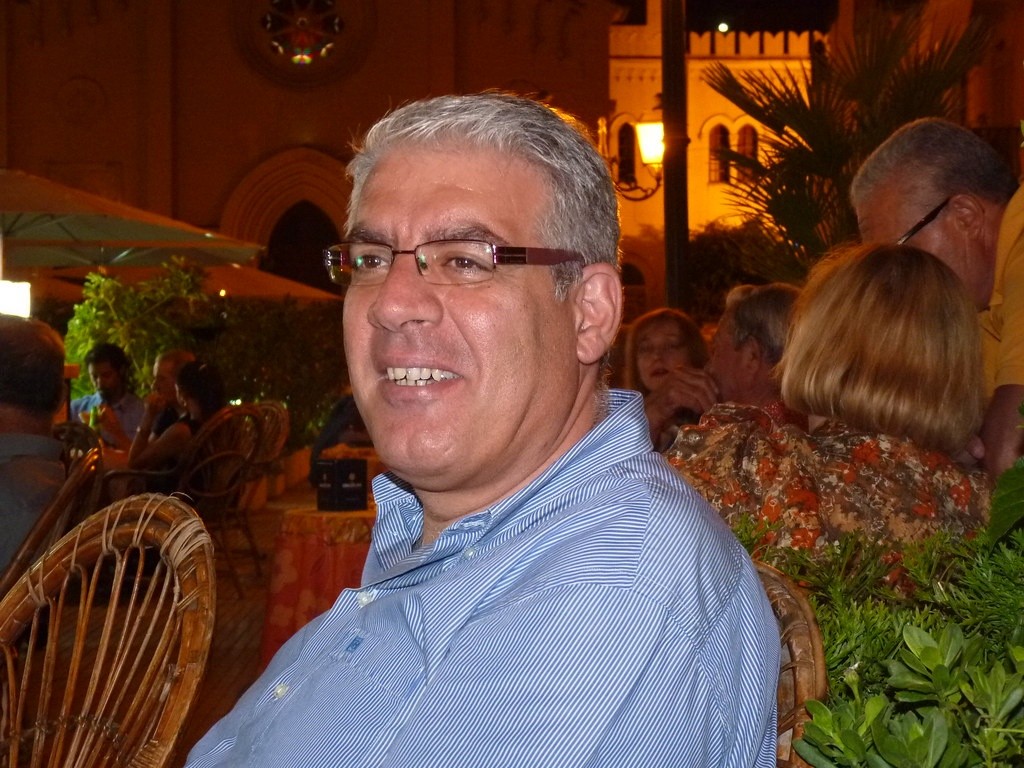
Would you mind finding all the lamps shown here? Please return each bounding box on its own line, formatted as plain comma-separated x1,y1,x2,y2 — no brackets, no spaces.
597,117,665,203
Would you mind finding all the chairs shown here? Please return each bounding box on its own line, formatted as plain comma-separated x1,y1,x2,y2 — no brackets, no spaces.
200,402,290,576
101,405,265,599
0,493,216,768
0,446,104,602
748,557,830,768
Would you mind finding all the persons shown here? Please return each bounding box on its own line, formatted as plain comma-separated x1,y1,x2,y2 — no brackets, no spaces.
175,85,783,768
0,313,230,584
622,108,1023,597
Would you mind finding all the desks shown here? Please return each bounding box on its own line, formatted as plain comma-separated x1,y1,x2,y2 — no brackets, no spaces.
255,502,377,675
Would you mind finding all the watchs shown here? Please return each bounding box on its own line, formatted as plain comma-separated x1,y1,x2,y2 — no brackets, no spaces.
136,425,150,437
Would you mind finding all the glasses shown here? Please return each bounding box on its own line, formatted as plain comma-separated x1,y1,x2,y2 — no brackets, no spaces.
322,239,582,288
